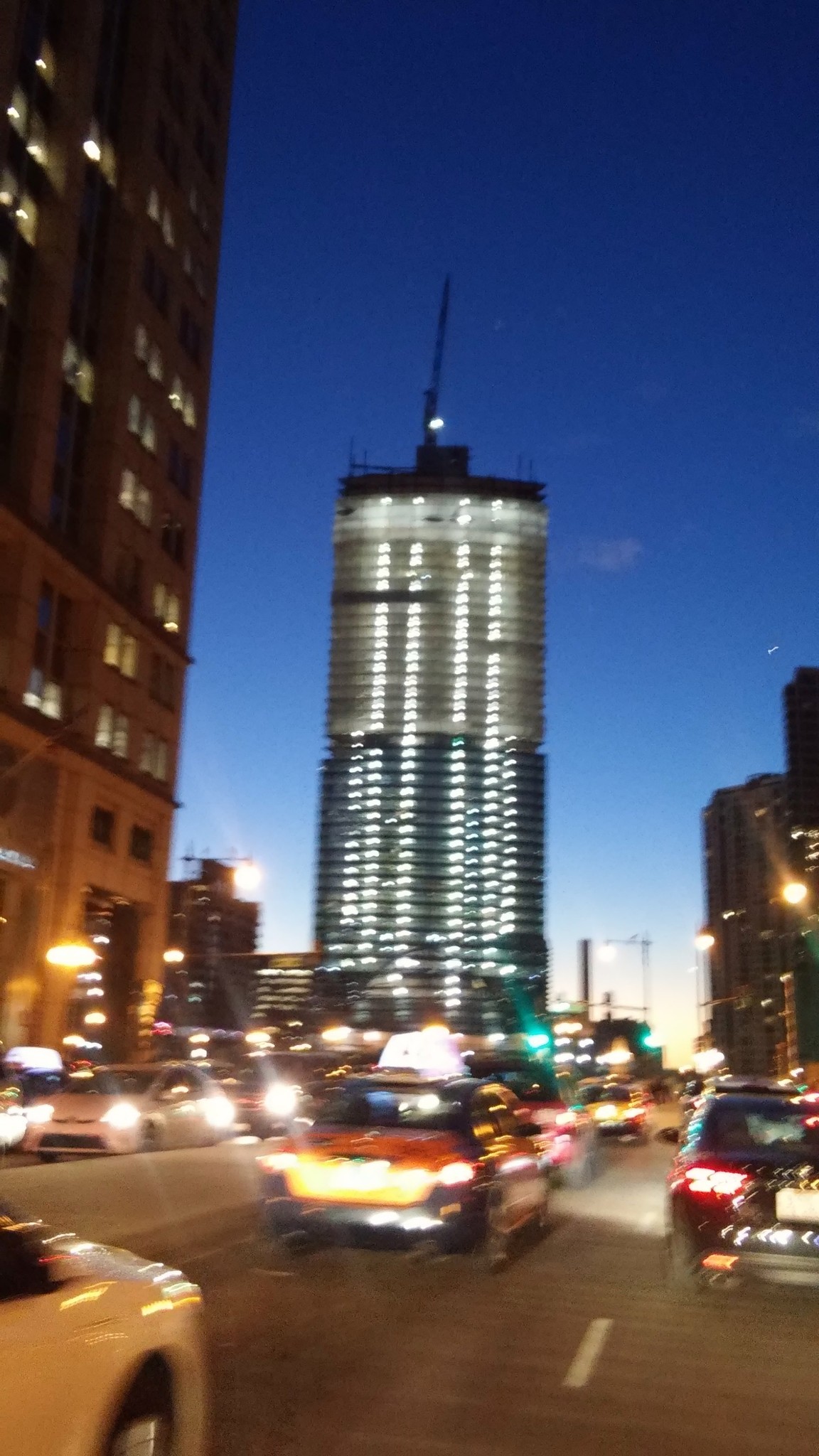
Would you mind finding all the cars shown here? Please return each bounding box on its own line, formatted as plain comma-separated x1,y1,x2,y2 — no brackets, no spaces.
21,1063,235,1161
2,1045,64,1088
150,1010,818,1167
457,1049,600,1189
258,1064,552,1260
0,1195,210,1456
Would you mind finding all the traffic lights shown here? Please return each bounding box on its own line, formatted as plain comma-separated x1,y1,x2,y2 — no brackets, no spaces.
523,1029,551,1054
642,1034,663,1051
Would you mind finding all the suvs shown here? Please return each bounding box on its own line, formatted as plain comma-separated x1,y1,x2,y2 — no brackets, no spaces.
652,1085,819,1294
